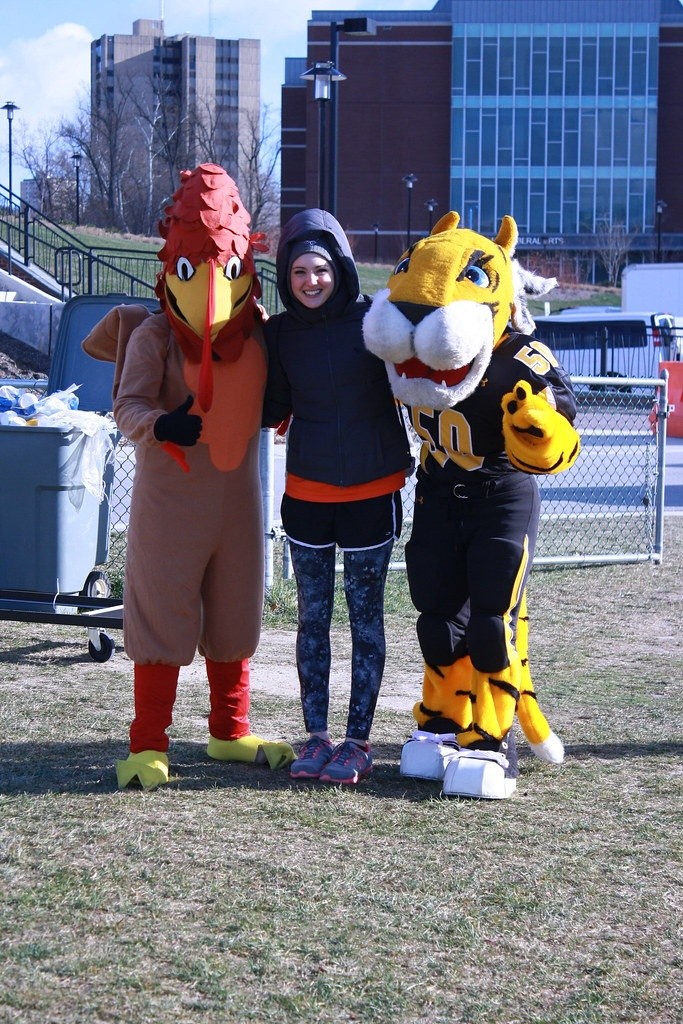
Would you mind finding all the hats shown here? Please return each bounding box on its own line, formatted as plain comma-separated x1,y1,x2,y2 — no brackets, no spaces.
287,238,339,310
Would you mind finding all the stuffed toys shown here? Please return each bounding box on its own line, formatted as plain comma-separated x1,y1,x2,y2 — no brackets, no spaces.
81,162,296,792
362,211,580,799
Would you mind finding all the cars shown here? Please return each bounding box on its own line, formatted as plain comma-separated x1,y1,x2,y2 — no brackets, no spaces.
550,305,622,314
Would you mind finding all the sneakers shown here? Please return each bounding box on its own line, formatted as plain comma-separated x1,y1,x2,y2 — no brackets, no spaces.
290,736,336,778
320,742,374,784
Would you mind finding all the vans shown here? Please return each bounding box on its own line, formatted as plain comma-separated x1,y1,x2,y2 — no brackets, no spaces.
510,312,677,394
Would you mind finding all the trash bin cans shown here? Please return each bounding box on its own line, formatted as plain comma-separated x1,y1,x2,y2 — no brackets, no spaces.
0,293,159,614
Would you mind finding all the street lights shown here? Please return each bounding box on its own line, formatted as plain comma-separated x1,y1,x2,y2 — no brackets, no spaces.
401,172,419,249
424,199,439,235
654,200,668,263
0,101,20,215
70,154,83,225
371,224,382,262
299,60,347,212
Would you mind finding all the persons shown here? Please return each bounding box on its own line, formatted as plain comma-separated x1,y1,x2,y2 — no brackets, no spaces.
261,210,418,784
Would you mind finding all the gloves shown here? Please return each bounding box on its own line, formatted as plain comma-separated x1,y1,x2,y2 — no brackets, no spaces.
154,395,203,446
